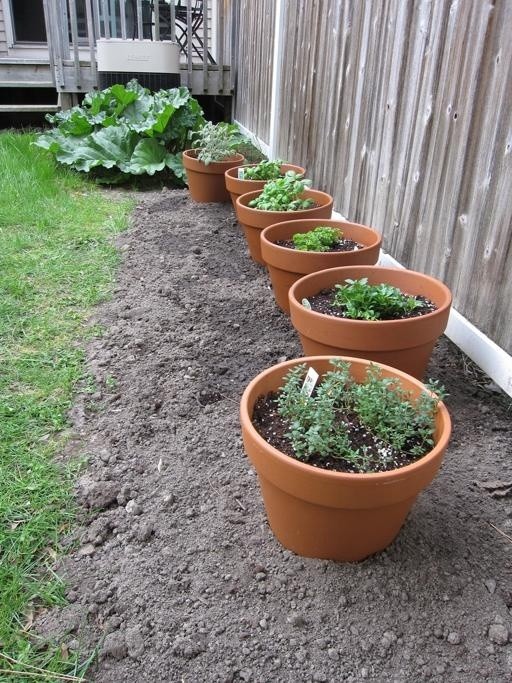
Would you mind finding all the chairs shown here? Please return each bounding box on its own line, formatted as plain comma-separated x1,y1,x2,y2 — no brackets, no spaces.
130,0,216,64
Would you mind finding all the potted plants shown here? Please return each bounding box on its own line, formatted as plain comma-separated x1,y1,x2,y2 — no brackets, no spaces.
260,218,381,316
288,265,453,382
183,122,252,204
225,161,304,217
236,171,333,264
239,355,452,563
